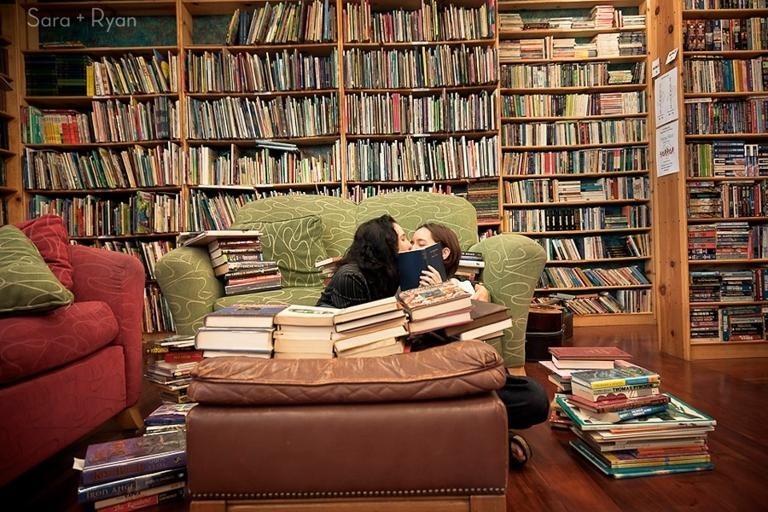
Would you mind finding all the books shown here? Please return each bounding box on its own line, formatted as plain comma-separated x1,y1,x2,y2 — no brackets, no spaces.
535,345,720,479
14,0,766,363
69,333,206,512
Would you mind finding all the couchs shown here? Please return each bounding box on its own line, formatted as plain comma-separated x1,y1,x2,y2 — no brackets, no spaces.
154,193,548,379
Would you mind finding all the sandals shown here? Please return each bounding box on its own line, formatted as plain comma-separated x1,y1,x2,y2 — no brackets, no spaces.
508,430,533,471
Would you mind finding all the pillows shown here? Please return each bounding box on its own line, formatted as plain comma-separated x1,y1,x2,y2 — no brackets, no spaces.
0,214,76,314
228,215,330,289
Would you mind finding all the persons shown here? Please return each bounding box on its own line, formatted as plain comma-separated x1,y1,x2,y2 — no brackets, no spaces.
394,222,551,464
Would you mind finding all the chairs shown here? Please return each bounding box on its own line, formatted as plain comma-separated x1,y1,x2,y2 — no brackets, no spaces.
1,241,146,495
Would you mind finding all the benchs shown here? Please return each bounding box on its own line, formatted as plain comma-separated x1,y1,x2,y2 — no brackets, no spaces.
184,340,510,512
